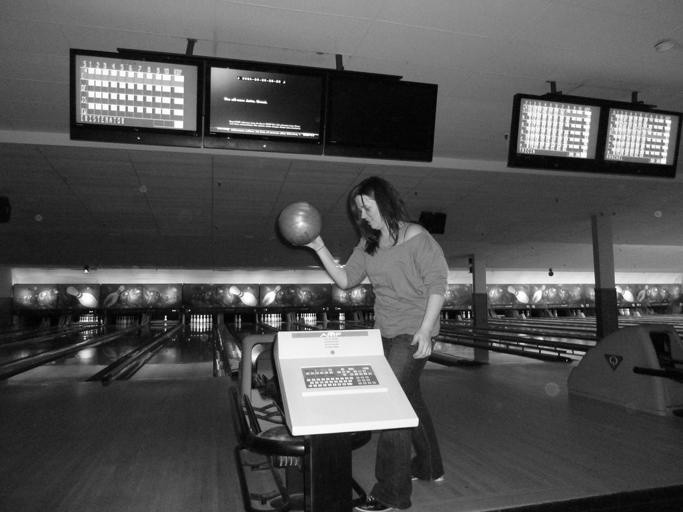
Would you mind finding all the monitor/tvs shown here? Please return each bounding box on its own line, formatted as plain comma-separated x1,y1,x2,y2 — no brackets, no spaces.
324,67,438,163
506,92,604,172
600,97,683,179
67,46,202,149
204,56,325,156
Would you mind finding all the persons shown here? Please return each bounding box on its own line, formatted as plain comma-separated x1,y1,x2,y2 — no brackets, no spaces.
299,175,449,512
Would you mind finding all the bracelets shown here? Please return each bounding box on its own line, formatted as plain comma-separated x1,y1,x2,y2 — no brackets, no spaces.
315,244,326,254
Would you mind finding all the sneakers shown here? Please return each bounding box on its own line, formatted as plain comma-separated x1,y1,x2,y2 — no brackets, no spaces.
354,496,398,512
411,473,444,482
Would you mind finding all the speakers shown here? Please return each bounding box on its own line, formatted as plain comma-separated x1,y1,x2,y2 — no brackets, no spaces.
0,196,11,224
419,210,446,235
